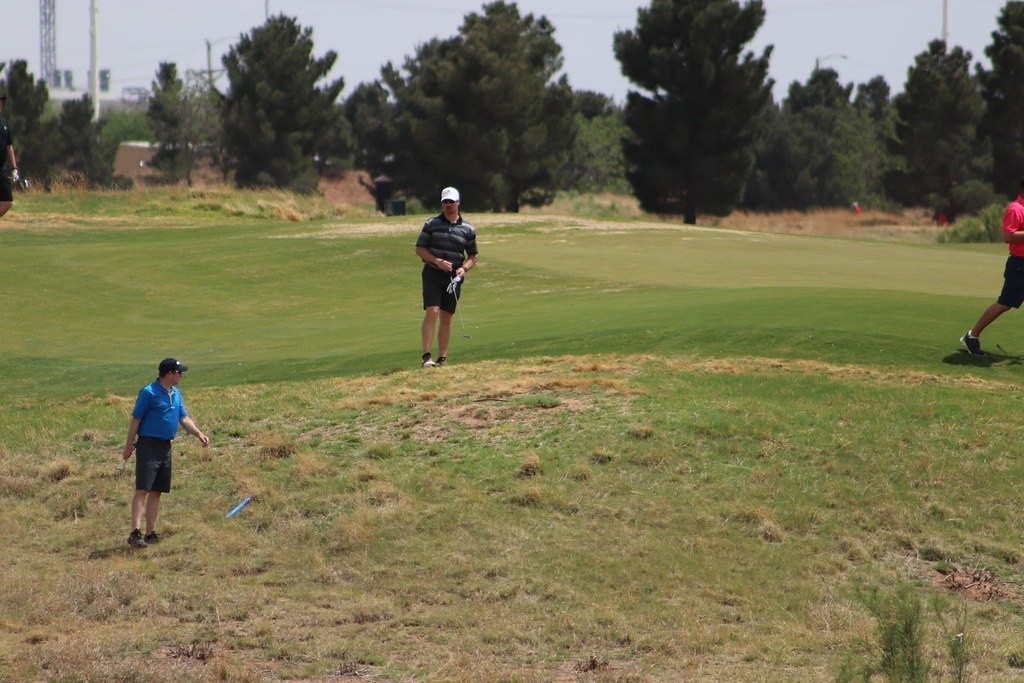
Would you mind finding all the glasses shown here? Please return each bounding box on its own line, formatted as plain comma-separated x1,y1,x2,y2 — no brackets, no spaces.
442,200,455,204
169,371,182,374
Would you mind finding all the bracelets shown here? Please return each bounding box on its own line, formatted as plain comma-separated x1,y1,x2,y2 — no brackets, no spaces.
462,266,467,272
12,168,17,170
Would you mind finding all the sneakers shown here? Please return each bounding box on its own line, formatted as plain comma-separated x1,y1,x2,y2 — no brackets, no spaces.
143,531,160,545
960,331,986,357
127,528,146,548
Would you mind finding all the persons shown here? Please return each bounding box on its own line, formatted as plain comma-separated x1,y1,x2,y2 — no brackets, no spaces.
851,201,862,215
415,186,478,368
960,186,1024,357
0,90,19,218
122,358,209,549
932,195,956,229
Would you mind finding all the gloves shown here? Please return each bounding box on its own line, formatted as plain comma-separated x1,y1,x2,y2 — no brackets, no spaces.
11,169,19,183
446,277,460,294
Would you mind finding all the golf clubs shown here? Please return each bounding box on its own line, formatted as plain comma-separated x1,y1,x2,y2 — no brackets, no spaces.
448,271,472,340
8,175,29,188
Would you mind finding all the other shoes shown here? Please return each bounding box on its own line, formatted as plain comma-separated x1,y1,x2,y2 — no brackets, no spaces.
437,357,447,366
422,354,435,366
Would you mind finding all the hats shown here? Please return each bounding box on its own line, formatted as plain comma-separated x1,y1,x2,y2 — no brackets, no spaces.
158,357,188,374
441,187,460,201
